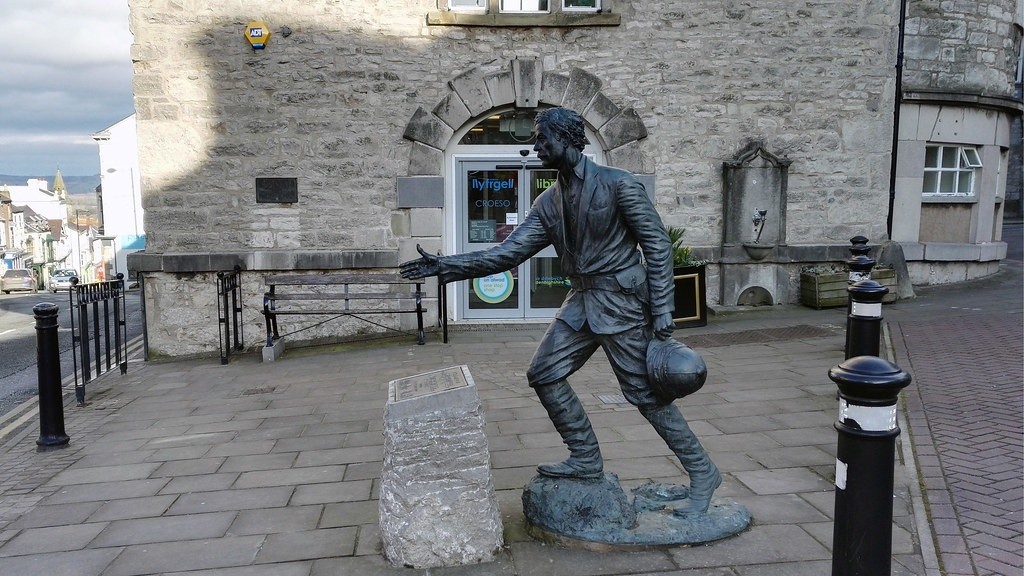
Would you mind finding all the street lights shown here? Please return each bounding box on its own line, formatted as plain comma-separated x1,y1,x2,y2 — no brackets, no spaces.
105,166,138,238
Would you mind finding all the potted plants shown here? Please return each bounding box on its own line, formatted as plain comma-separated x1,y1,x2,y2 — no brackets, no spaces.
800,264,848,310
665,225,707,329
871,263,899,302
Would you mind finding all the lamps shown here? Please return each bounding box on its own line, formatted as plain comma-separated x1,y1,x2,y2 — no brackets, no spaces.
753,210,767,244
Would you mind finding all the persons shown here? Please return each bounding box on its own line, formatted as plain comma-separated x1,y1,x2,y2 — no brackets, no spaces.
398,107,722,517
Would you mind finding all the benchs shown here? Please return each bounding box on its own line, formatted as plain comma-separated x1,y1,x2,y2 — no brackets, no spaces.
260,274,428,345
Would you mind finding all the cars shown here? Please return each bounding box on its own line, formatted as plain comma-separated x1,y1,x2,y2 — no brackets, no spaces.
0,269,37,295
48,268,80,294
